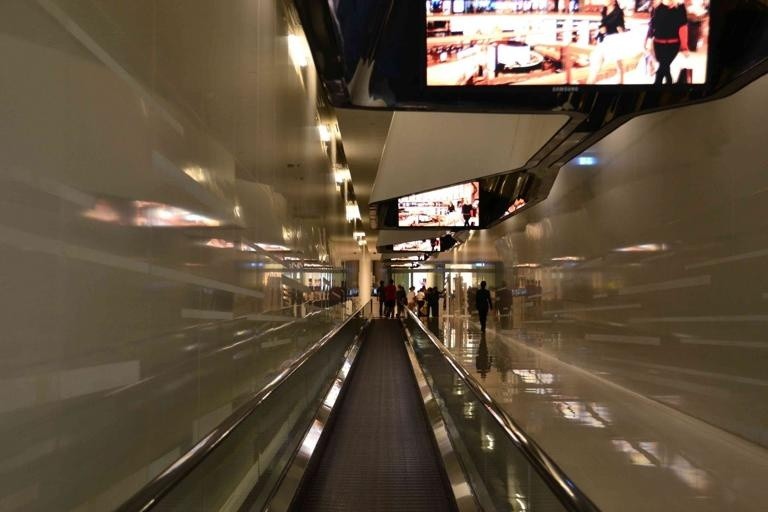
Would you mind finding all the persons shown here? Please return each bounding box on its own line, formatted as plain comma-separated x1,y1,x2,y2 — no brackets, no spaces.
643,1,689,85
462,199,472,227
377,279,449,319
586,0,628,86
474,333,494,382
448,273,543,333
447,201,456,226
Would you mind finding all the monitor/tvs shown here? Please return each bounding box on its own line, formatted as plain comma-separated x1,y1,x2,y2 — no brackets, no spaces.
389,235,443,268
396,179,483,229
419,0,720,94
